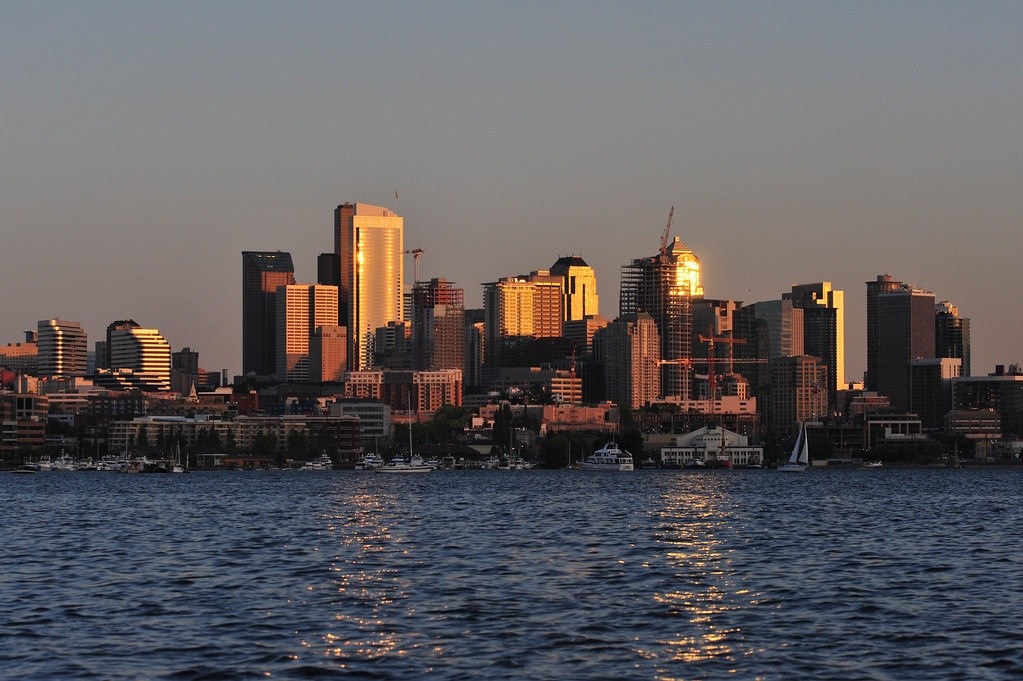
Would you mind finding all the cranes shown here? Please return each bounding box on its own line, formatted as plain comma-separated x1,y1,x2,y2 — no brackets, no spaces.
659,207,676,255
404,248,423,282
699,334,747,412
654,357,769,413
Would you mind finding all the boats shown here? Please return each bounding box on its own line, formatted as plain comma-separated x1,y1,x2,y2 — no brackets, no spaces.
25,439,185,474
434,453,533,471
863,457,883,467
714,421,733,469
575,444,634,473
686,456,705,468
303,452,384,470
747,464,761,469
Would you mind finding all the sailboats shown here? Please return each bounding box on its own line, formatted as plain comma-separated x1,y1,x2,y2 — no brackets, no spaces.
376,390,434,473
778,422,810,472
